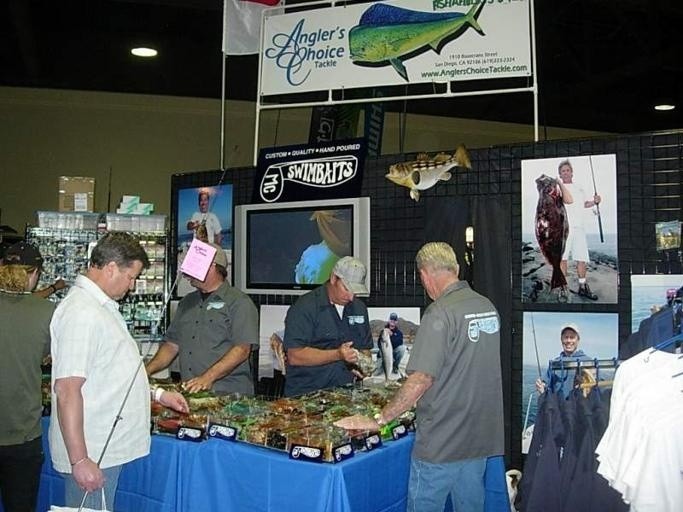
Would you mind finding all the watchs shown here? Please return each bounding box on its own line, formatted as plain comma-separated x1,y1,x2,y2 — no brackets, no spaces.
374,412,386,427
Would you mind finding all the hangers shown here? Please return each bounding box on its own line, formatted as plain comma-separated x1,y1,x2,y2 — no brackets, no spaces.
572,358,587,400
610,357,622,379
543,360,556,395
556,360,566,404
644,296,682,394
588,356,604,400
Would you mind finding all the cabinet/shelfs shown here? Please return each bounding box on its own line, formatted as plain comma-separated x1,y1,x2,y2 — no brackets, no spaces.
26,225,166,338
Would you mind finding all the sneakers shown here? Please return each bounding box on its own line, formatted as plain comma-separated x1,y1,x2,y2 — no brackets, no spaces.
579,284,598,301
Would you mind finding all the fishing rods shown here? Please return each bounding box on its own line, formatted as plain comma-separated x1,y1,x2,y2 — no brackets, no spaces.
78,145,239,512
531,312,545,394
590,155,604,243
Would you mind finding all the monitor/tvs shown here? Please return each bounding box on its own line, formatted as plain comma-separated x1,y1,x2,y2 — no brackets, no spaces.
234,196,371,297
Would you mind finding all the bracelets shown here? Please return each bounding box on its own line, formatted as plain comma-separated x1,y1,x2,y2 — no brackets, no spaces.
71,457,88,465
152,387,164,403
50,285,56,293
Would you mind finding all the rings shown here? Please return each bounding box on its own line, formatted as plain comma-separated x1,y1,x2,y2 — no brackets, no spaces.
201,384,204,387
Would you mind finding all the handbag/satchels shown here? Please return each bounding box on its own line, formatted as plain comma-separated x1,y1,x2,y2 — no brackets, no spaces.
48,486,110,512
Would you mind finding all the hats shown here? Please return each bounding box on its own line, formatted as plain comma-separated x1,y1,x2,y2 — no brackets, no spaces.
206,243,227,268
0,240,46,272
390,312,397,321
333,255,368,296
560,325,580,339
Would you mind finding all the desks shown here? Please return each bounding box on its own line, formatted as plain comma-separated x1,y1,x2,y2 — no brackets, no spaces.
37,379,508,511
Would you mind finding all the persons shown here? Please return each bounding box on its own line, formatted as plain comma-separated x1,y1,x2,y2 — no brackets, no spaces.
145,243,260,399
0,244,64,511
187,192,221,244
50,230,189,510
333,242,504,512
0,226,65,298
557,160,601,301
285,255,375,396
378,313,404,351
536,324,596,396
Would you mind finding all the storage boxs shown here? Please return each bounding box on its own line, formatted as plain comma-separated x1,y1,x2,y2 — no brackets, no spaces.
56,176,97,213
